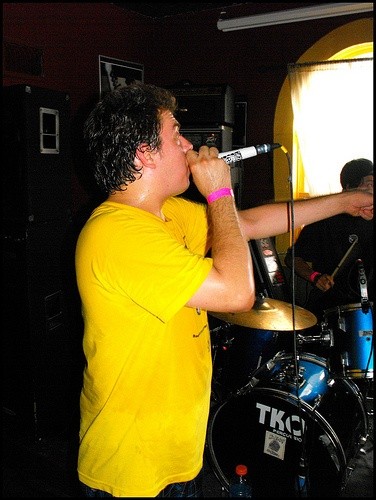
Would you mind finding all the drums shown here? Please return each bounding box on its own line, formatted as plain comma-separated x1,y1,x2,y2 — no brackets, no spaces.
210,349,370,500
322,301,375,384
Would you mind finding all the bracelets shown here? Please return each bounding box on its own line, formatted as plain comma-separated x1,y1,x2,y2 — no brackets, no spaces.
309,272,322,286
206,188,234,205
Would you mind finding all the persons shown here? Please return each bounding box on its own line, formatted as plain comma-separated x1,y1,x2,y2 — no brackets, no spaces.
74,80,376,500
284,158,376,330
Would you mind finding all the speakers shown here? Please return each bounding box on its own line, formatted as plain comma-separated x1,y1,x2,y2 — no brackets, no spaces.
175,125,233,205
0,223,84,433
0,85,70,224
166,83,234,124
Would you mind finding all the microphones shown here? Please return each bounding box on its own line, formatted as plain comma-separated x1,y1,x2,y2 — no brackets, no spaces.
217,142,279,165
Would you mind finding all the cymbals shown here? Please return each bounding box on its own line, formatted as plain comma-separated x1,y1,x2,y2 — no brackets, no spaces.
209,296,319,333
